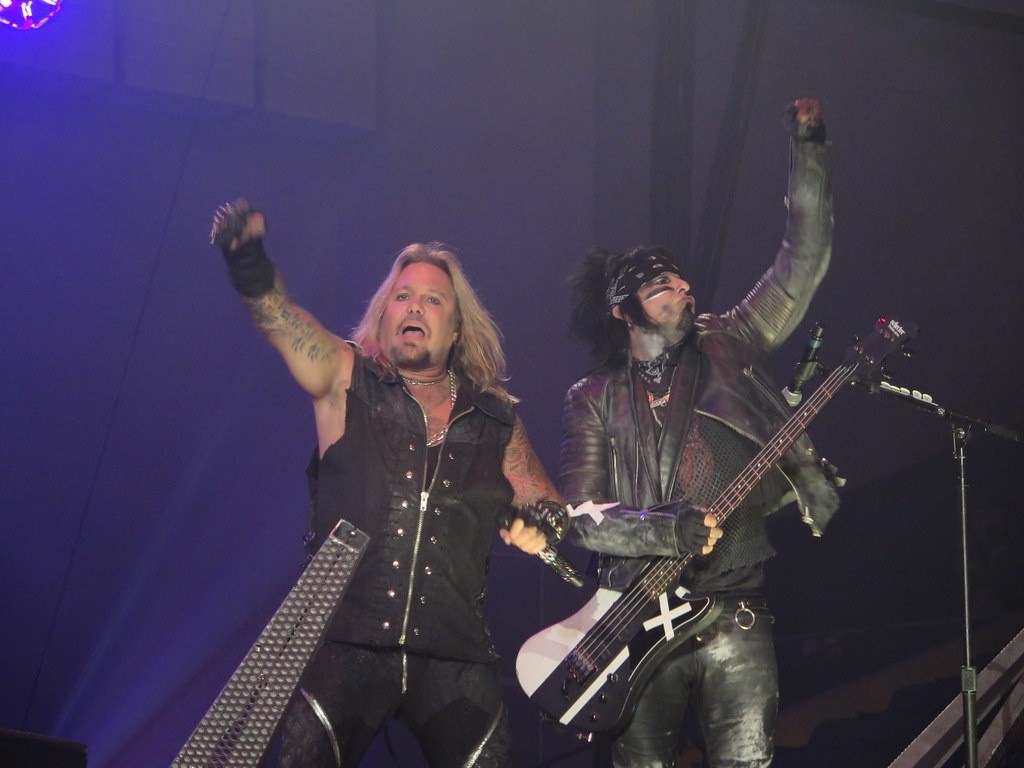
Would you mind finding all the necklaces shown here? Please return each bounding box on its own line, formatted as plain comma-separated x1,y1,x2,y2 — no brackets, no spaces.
398,376,440,387
634,349,680,427
426,370,457,450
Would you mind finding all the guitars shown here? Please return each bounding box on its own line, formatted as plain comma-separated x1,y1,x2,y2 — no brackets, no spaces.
512,316,926,745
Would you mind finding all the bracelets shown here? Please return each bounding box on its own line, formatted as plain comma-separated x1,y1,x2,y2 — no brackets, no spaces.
533,499,570,546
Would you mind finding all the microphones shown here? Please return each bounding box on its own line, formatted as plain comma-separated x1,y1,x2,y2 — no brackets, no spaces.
782,322,825,407
496,506,585,587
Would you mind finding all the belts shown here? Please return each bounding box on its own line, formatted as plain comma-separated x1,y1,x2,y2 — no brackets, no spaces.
718,593,768,608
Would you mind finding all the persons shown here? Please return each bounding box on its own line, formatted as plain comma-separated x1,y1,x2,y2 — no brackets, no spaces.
211,196,570,768
558,96,835,768
